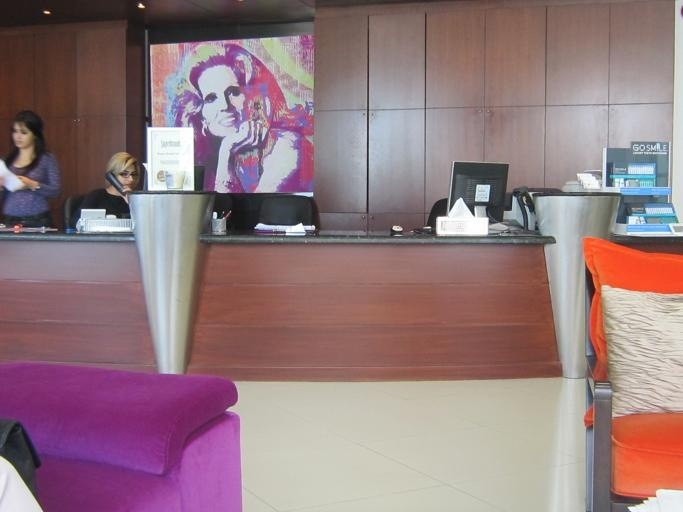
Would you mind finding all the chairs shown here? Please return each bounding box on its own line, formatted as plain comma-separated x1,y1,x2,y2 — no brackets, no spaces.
580,243,683,512
256,194,319,232
63,194,83,229
426,197,447,232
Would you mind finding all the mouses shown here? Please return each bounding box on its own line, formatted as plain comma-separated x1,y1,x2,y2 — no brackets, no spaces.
391,224,403,232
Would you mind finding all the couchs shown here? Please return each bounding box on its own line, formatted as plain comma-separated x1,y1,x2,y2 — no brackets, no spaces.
0,356,241,512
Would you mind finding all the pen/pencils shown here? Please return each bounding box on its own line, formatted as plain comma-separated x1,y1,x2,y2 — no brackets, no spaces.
221,210,231,220
217,212,225,220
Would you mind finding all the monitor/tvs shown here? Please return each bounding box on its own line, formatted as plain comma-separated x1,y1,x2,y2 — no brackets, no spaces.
445,159,510,223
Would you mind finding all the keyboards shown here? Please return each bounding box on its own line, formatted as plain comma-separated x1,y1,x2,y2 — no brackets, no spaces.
413,227,435,233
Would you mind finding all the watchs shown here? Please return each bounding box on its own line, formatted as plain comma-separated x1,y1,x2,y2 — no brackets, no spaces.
35,183,40,190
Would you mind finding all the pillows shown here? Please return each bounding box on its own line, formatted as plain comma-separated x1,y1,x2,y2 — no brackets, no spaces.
578,237,683,426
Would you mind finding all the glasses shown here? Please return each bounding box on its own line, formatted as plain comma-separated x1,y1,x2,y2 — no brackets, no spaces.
119,169,138,177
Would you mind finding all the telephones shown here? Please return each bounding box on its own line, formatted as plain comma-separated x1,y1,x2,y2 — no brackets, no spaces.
104,171,127,196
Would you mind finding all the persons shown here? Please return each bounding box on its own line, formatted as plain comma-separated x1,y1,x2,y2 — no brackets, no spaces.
0,111,62,228
171,44,314,192
69,151,140,230
